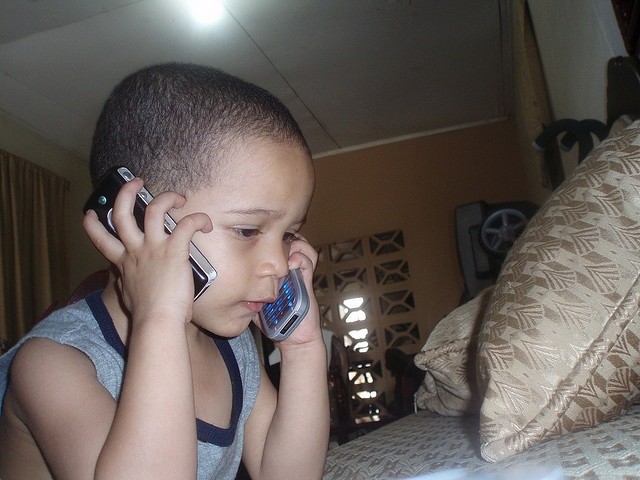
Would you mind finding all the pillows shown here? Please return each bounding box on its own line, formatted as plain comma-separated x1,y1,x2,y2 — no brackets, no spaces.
472,118,640,461
413,285,496,415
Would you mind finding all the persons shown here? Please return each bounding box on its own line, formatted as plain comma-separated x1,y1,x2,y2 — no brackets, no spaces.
1,61,332,480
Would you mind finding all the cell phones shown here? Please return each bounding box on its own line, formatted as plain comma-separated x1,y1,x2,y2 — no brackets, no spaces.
259,269,310,341
82,166,218,301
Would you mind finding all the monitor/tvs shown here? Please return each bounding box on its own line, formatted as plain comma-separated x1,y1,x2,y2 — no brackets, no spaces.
453,200,534,299
